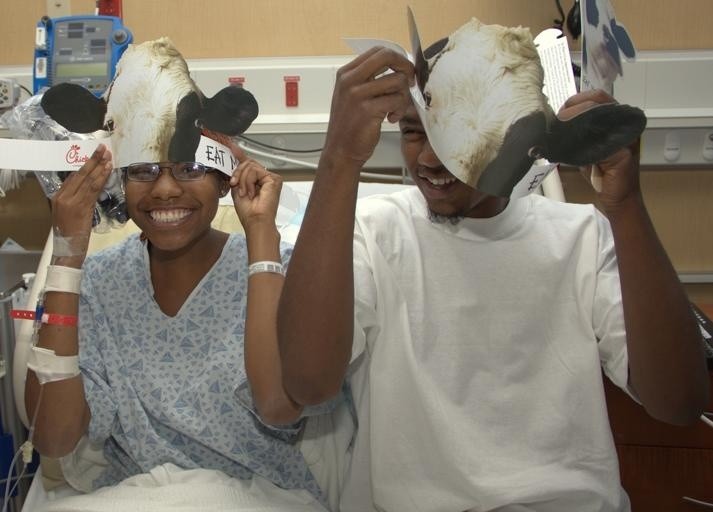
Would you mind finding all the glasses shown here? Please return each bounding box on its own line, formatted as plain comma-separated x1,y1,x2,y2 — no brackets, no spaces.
124,162,209,181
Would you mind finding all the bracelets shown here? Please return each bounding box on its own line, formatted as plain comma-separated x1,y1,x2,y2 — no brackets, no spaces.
34,307,81,329
247,261,285,276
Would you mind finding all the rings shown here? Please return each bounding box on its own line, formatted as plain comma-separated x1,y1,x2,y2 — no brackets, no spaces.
265,167,266,171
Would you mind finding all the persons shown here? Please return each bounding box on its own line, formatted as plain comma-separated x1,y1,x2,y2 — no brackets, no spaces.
24,143,328,512
276,38,710,512
